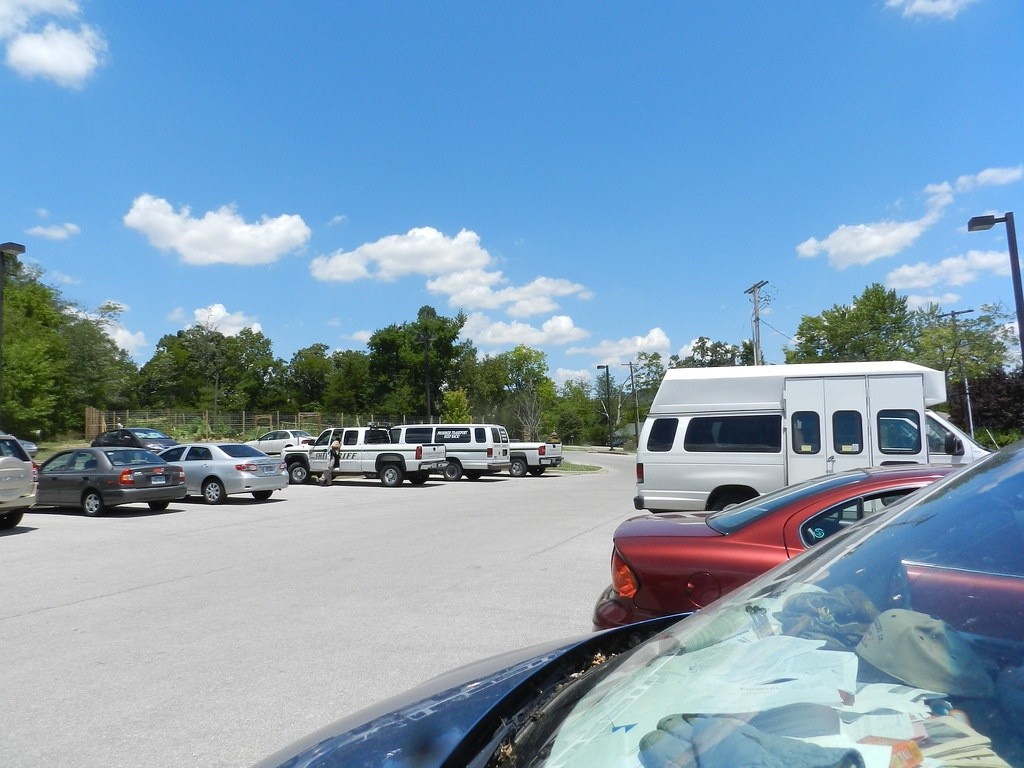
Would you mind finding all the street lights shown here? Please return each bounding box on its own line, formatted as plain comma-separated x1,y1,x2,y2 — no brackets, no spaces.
967,212,1023,364
597,364,616,450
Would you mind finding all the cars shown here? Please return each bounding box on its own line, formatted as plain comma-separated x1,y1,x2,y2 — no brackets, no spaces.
0,429,38,457
606,438,627,446
34,446,187,518
154,443,290,505
590,464,1024,634
91,428,181,457
257,437,1024,768
244,429,318,456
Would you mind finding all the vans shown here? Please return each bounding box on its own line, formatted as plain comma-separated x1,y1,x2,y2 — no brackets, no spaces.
388,423,513,481
634,361,1002,513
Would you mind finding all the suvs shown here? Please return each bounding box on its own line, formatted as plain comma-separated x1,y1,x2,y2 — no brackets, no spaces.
0,433,40,530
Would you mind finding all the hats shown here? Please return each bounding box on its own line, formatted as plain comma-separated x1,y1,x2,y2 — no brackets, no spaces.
860,607,996,699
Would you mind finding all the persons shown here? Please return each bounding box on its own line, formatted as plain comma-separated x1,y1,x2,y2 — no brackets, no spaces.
318,438,341,486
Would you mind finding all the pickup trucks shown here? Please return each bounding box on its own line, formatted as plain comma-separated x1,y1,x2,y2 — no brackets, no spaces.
508,439,565,477
281,421,449,487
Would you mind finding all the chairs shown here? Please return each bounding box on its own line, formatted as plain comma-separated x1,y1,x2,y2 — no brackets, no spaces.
85,459,97,469
113,461,124,465
188,450,212,458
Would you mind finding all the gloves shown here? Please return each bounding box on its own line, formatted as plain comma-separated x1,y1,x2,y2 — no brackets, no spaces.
639,713,865,768
